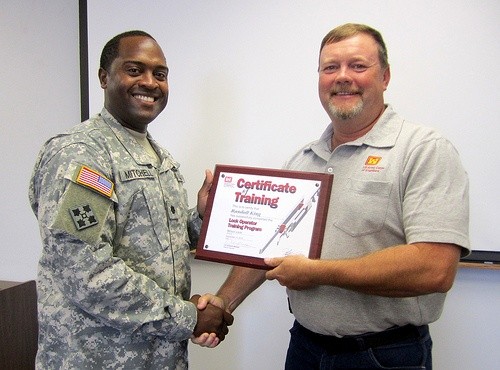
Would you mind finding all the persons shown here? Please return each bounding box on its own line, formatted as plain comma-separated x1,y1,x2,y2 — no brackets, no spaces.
191,22,472,370
28,30,235,370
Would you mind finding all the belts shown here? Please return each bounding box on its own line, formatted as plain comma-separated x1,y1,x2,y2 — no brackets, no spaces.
292,319,429,354
